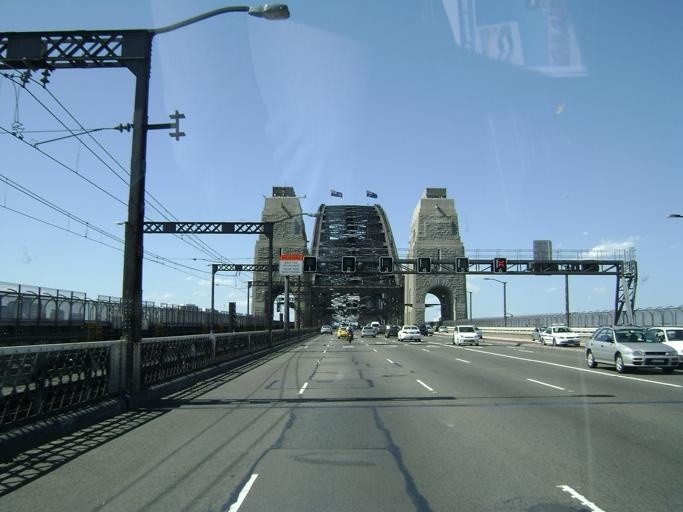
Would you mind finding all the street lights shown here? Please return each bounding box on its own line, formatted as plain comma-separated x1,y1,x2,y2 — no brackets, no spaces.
117,1,293,398
267,210,324,345
482,277,506,326
664,213,683,219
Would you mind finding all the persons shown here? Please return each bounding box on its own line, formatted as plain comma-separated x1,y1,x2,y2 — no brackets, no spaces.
347,326,352,333
345,323,353,341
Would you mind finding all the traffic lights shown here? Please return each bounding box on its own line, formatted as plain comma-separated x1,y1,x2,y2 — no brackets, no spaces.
493,257,508,272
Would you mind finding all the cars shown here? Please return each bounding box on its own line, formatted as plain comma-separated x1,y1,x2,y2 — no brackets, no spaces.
530,322,682,374
320,321,484,347
